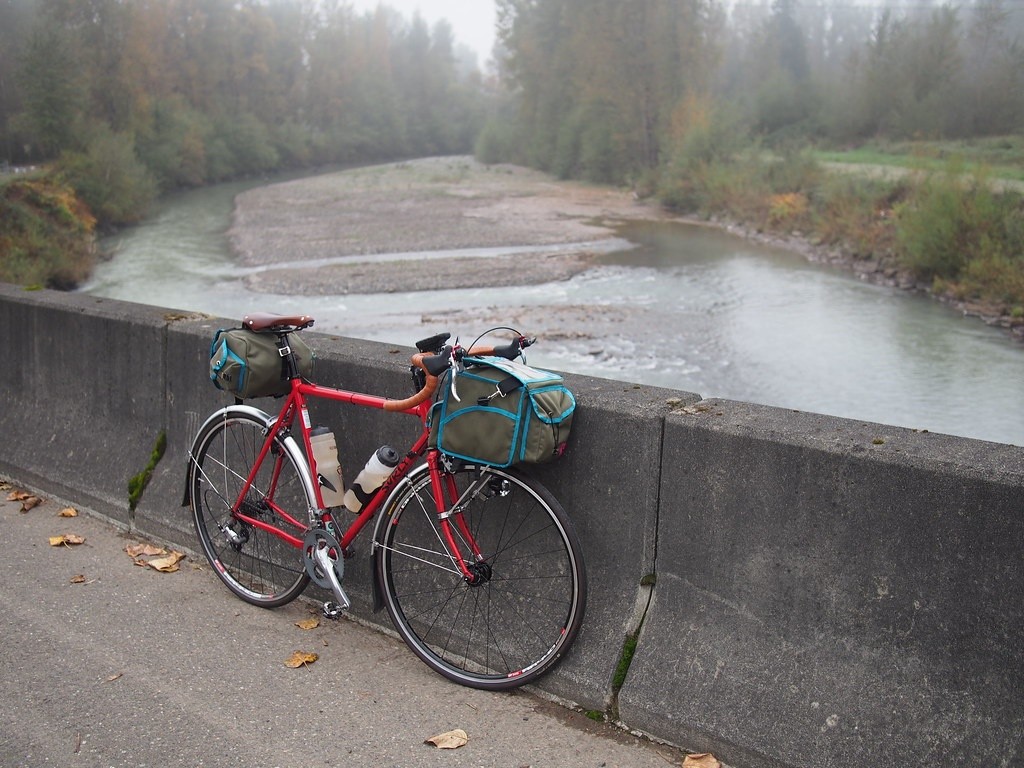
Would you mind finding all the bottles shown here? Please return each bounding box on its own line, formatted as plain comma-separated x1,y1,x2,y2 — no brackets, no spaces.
309,422,345,508
342,444,400,513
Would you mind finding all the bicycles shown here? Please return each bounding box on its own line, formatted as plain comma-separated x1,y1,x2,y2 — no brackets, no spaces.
180,313,589,691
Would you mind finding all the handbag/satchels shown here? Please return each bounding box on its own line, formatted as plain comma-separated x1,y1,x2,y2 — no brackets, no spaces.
209,327,312,400
